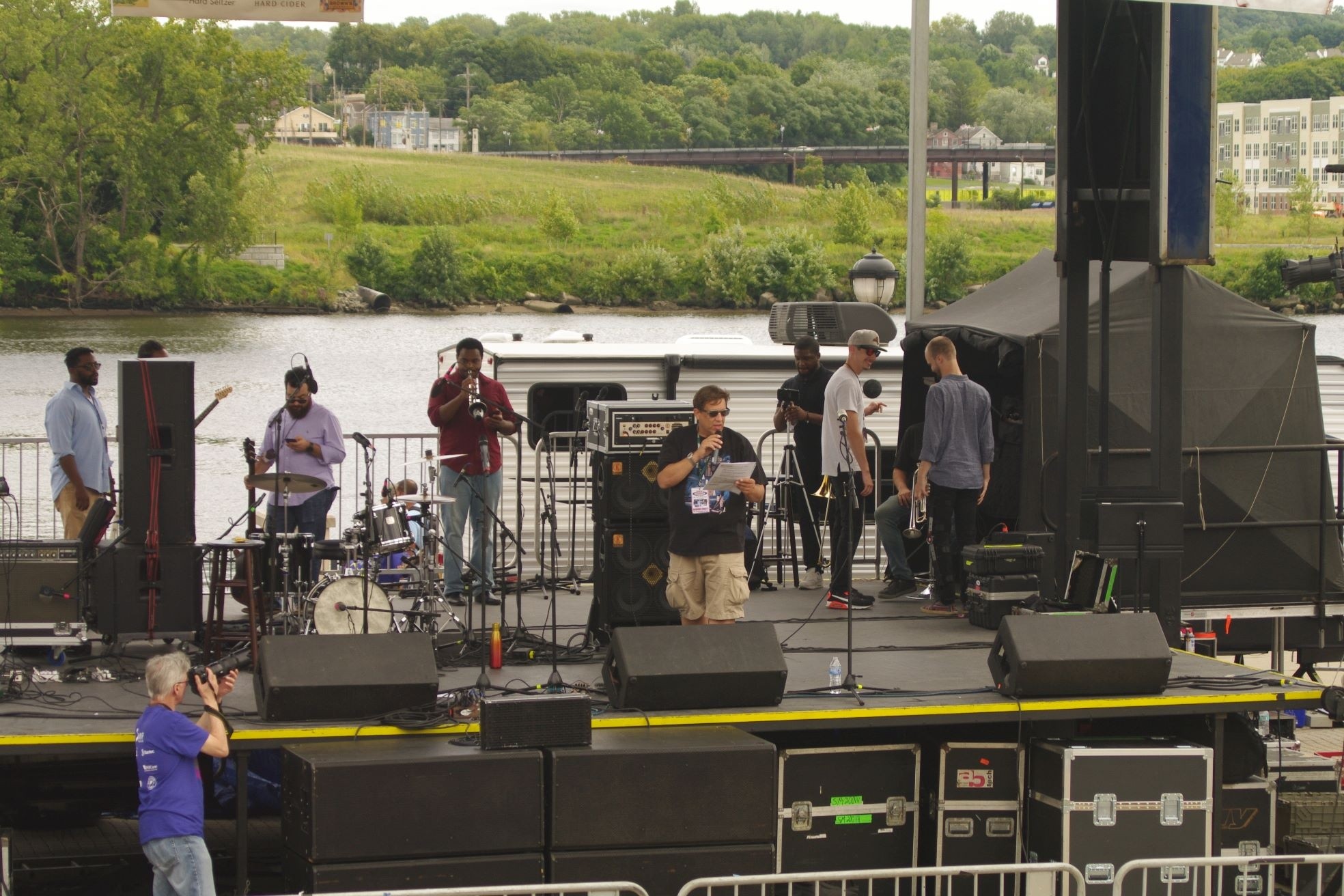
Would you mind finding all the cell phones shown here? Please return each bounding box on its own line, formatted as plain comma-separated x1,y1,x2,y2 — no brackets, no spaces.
285,438,298,443
777,388,800,403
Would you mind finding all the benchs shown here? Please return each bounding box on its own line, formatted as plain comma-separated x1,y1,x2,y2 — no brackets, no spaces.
779,447,896,578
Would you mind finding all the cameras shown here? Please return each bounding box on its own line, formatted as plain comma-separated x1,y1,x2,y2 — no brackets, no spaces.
188,642,256,691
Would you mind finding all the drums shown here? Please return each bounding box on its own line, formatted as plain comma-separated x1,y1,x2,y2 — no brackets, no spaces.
301,574,394,635
247,531,316,598
345,526,370,560
352,500,416,557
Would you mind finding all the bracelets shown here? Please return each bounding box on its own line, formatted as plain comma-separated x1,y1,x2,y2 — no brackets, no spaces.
805,411,809,424
306,442,314,454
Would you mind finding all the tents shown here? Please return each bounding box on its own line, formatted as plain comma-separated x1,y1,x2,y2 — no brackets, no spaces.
894,247,1344,652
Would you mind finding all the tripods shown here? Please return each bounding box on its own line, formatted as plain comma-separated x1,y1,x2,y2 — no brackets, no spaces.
399,380,599,663
747,408,826,588
250,477,313,635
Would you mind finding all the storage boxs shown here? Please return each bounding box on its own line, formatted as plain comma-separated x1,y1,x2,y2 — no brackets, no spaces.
776,746,1276,896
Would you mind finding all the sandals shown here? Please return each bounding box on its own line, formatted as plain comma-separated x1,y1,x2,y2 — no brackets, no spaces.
920,604,959,615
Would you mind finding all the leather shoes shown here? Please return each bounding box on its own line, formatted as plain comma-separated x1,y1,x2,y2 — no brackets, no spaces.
446,593,466,605
474,590,501,605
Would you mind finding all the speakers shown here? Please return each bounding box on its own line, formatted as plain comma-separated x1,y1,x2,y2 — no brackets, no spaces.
249,633,437,718
0,540,85,629
593,524,681,626
90,543,202,641
114,357,194,543
1083,498,1182,644
990,613,1171,695
605,622,788,711
591,448,675,525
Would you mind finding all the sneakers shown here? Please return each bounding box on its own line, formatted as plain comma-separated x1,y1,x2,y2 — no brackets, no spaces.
799,567,824,589
825,587,873,610
879,580,917,598
852,588,876,602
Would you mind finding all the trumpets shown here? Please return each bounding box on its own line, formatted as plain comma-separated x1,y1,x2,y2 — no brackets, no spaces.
902,462,927,539
468,369,488,419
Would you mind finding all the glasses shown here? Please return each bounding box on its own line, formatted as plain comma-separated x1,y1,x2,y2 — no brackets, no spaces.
859,346,880,357
75,363,101,371
699,408,730,418
285,392,310,404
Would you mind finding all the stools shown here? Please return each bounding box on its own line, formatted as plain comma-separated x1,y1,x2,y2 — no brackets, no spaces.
204,539,268,665
309,540,362,593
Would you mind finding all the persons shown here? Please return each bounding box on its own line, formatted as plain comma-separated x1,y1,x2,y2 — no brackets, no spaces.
138,339,170,357
875,421,934,598
135,651,238,896
914,337,995,615
242,366,346,614
657,384,769,625
378,479,430,589
427,338,518,607
774,336,837,589
822,330,888,608
45,346,117,541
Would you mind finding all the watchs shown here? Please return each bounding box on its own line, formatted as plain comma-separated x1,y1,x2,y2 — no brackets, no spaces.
687,452,696,467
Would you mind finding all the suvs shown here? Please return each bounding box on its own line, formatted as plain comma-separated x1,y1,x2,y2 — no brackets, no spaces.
952,143,980,154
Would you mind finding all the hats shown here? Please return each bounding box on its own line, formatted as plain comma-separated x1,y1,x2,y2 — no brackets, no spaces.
848,329,887,353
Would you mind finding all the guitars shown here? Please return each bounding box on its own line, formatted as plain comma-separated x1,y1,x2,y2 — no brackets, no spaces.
230,436,274,610
194,384,233,428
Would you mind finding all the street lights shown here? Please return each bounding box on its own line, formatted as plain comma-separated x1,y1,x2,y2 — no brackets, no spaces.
1015,155,1024,198
783,153,796,186
503,129,511,156
687,127,692,151
596,129,604,153
779,124,785,146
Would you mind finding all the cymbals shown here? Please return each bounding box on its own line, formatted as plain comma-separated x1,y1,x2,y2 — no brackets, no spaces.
397,454,469,467
393,493,458,506
246,472,328,493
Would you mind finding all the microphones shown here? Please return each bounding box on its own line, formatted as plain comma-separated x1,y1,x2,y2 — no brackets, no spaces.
39,583,79,599
336,602,346,612
405,513,430,522
431,361,459,397
574,392,588,414
381,478,388,497
351,433,375,451
304,355,317,394
453,463,468,487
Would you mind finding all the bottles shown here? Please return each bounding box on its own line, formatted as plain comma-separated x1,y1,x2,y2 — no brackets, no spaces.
1258,711,1269,736
490,623,501,668
829,656,841,694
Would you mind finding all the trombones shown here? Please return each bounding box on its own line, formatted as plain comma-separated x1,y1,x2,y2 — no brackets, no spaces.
810,474,837,569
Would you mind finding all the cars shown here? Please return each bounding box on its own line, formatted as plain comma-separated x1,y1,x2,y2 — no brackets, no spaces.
789,146,814,157
